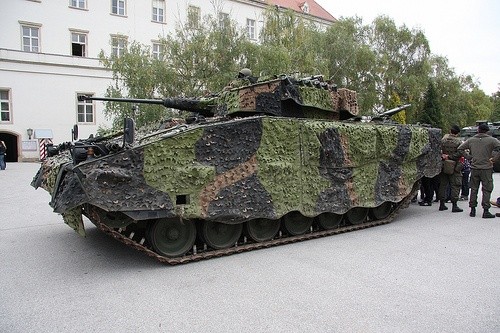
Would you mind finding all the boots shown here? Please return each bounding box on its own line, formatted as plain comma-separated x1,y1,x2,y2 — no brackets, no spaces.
483,209,495,218
439,202,448,211
452,203,463,212
469,206,475,217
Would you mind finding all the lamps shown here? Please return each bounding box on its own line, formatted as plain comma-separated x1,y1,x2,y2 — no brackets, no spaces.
27,127,33,139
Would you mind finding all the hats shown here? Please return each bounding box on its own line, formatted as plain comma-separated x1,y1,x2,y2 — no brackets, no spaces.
453,125,460,132
481,123,489,131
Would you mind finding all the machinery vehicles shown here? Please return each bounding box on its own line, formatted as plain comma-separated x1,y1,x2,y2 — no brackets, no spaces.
30,74,443,264
456,120,500,173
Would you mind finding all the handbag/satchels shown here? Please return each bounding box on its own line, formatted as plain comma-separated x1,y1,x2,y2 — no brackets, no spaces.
444,160,455,174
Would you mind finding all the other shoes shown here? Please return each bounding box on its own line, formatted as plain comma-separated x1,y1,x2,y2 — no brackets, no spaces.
459,195,468,201
428,202,432,206
490,200,500,208
421,193,424,199
411,195,417,202
433,198,440,202
496,213,500,217
418,201,424,205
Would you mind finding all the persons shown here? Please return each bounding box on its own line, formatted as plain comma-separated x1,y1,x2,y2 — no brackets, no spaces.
438,125,463,212
491,197,500,208
459,158,471,200
412,177,439,206
458,124,500,218
0,141,7,170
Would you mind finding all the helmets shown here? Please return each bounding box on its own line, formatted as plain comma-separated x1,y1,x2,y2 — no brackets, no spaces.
238,67,252,77
